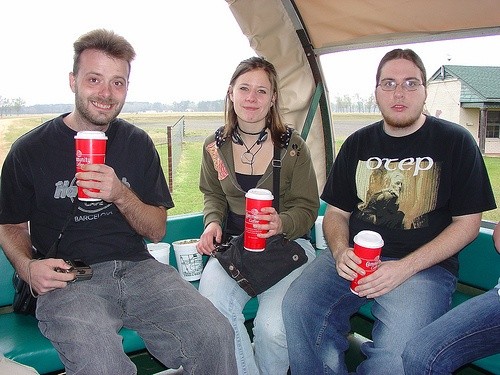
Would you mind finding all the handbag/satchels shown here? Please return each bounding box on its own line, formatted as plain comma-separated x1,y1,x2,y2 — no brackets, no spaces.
214,225,309,297
12,245,51,317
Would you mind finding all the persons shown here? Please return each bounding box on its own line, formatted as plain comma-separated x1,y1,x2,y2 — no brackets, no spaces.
403,221,500,375
197,57,321,375
0,29,238,375
280,48,497,375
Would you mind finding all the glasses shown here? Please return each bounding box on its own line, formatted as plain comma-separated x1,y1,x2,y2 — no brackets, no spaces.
377,80,423,91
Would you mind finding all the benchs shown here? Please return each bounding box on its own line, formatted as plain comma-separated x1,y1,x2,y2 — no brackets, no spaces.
0,199,500,375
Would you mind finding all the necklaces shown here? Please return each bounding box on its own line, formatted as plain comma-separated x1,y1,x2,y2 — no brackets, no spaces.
235,124,269,165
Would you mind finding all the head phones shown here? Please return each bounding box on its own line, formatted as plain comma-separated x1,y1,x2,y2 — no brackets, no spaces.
232,123,269,145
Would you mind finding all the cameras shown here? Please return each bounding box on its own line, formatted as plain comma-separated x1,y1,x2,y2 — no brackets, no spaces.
55,261,93,284
